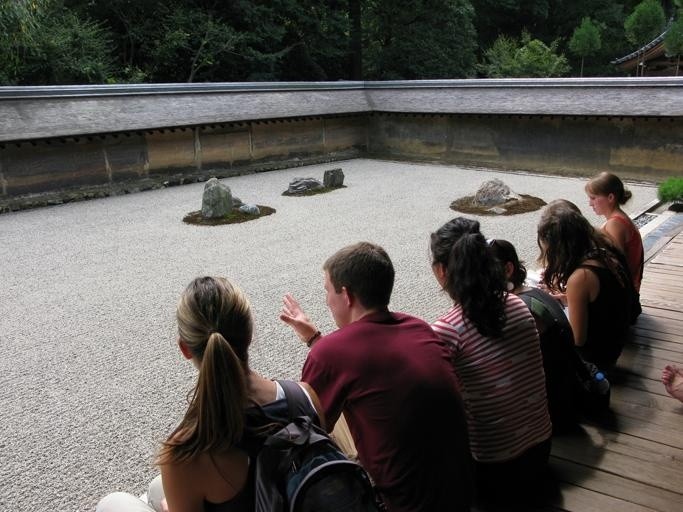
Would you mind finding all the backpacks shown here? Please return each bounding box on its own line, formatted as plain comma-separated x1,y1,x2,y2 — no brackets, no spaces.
238,380,382,511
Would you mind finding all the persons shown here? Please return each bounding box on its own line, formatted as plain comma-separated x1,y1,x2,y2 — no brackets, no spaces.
532,197,637,377
584,171,646,327
428,216,554,502
93,272,326,511
483,236,577,438
276,239,472,512
660,363,683,402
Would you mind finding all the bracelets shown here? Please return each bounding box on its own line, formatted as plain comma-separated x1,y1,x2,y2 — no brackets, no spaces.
306,330,320,348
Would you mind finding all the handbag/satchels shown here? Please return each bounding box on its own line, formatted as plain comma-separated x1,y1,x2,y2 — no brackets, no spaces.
574,356,614,425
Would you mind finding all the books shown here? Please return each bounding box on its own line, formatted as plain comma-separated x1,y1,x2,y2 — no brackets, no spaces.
526,266,565,290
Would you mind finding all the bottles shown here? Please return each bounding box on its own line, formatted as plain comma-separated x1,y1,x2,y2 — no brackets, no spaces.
594,372,612,396
